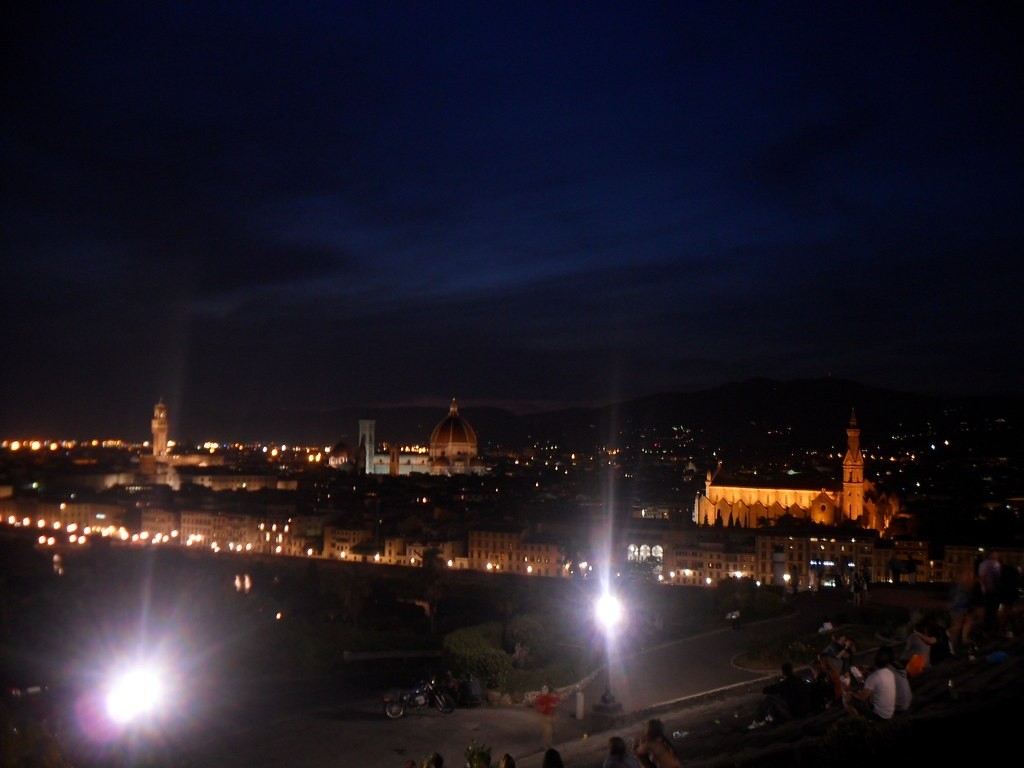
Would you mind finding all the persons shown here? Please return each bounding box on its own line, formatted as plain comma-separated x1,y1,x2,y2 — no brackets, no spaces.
501,752,516,768
426,669,480,709
476,752,493,768
746,602,1023,726
542,749,564,768
419,753,443,768
631,717,681,768
603,737,640,768
848,567,870,608
532,683,561,751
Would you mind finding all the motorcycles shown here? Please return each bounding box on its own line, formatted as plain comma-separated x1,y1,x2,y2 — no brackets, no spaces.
383,676,455,720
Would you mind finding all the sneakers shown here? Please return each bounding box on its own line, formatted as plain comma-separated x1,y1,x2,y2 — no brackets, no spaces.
765,714,778,726
747,720,767,730
1006,630,1014,637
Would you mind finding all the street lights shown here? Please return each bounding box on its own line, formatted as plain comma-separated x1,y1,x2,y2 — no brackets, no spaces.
601,599,617,703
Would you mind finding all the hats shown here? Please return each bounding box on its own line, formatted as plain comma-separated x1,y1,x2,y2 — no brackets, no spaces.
781,664,794,682
